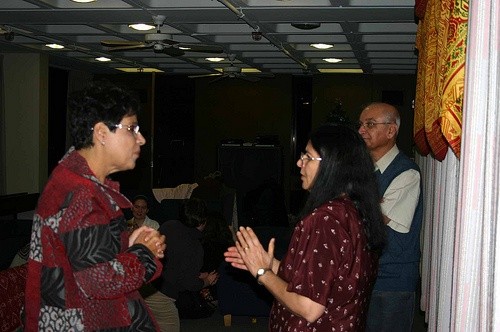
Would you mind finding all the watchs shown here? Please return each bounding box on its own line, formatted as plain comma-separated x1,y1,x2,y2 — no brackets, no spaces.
255,268,272,285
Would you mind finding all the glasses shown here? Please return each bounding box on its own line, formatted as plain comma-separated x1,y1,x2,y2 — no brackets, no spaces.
91,124,139,135
300,152,323,167
356,121,392,129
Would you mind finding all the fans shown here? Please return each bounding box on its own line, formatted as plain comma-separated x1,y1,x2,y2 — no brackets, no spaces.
188,50,275,81
98,13,224,60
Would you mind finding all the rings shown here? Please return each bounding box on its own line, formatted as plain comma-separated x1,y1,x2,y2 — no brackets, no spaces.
243,247,249,252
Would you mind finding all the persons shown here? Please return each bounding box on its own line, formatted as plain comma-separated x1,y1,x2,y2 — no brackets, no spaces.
127,194,159,233
25,85,166,332
359,102,423,332
223,125,386,332
156,201,215,317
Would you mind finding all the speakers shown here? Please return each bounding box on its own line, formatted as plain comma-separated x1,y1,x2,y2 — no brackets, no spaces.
213,145,284,228
